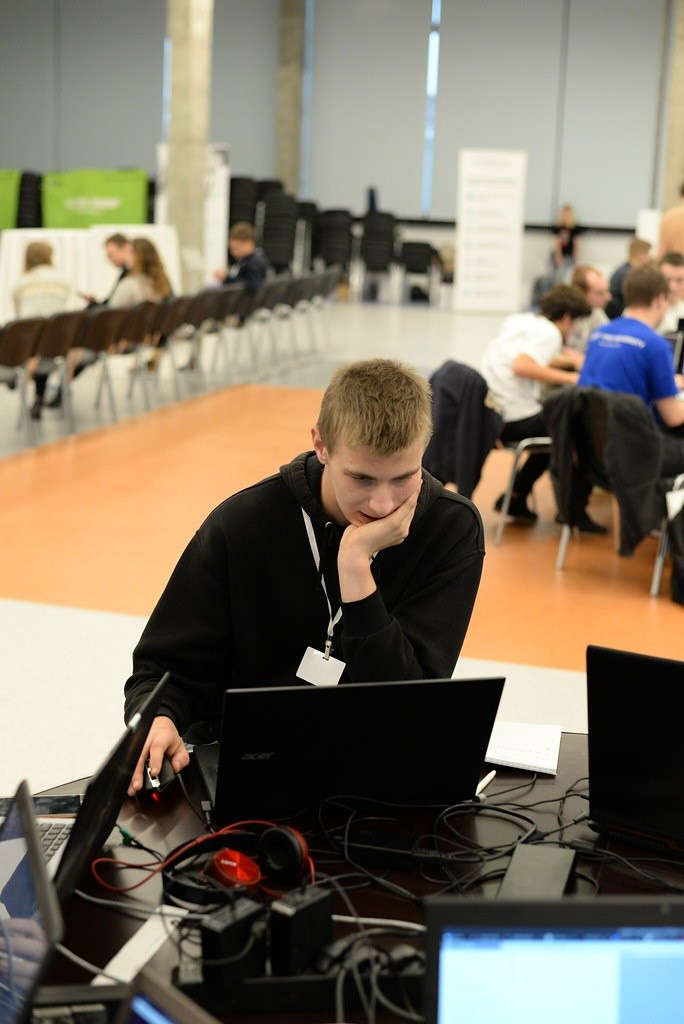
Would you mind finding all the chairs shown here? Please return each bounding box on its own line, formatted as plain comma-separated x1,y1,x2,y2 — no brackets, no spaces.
0,267,341,434
425,361,684,602
229,176,444,306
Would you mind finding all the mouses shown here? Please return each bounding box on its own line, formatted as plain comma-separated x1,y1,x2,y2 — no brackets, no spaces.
133,758,179,817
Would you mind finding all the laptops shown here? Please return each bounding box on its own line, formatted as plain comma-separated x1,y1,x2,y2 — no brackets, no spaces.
0,645,684,1024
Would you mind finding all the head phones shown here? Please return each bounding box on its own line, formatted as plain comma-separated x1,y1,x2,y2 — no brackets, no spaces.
162,823,309,908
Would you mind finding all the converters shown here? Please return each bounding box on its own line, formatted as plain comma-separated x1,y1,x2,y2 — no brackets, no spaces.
496,843,576,900
198,895,267,980
269,884,334,977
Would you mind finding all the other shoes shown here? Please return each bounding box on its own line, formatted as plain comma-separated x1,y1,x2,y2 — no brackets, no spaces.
495,492,537,521
31,405,42,418
556,507,606,532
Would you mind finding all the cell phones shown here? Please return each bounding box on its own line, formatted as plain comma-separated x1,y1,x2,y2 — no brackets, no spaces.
0,793,85,818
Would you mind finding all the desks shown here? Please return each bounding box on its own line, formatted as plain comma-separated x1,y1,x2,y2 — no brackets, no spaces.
34,757,684,1024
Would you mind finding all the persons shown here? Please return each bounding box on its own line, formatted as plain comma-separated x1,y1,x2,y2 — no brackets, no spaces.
123,359,484,795
0,221,275,421
466,179,684,604
